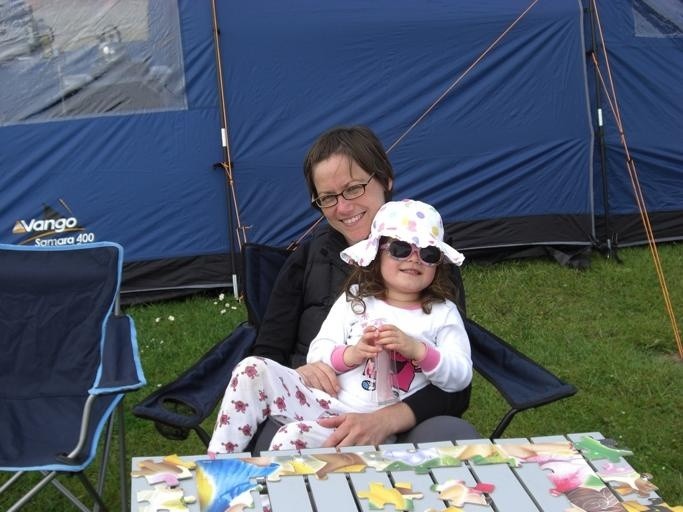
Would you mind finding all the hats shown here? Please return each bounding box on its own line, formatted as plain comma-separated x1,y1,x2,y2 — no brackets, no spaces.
339,199,467,267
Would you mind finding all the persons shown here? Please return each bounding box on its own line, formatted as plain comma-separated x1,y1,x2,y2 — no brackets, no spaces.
252,125,482,454
208,199,474,455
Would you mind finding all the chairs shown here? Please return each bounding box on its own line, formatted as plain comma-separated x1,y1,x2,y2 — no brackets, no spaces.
1,233,147,512
129,242,573,446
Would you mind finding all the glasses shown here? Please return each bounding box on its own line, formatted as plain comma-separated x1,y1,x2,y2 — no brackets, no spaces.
311,172,376,209
380,240,445,267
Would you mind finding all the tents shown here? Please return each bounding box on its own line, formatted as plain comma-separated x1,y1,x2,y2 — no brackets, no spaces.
0,0,683,306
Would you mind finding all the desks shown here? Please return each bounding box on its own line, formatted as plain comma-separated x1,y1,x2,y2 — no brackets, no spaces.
128,431,671,512
